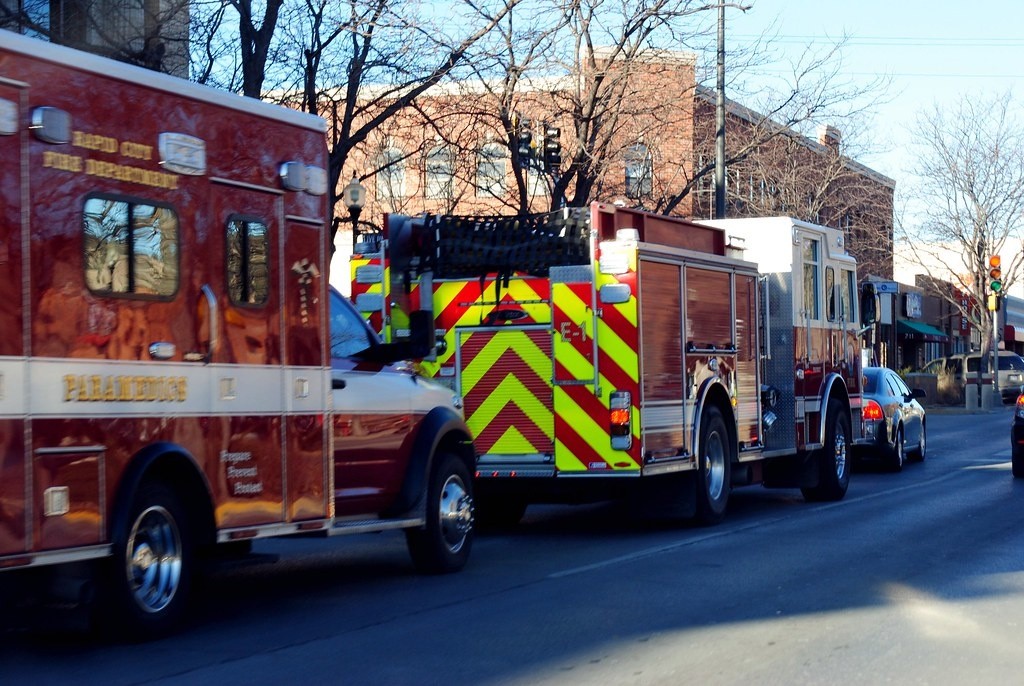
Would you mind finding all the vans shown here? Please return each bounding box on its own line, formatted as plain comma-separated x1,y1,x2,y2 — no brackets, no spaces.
922,350,1024,405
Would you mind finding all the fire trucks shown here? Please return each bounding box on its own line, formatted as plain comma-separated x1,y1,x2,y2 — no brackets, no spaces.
346,199,882,536
1,30,480,640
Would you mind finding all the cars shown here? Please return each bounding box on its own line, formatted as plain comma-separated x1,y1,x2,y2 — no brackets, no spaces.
849,367,928,474
1010,388,1024,481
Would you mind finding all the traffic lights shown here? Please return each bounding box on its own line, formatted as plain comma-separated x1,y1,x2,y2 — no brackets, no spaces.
989,254,1003,294
